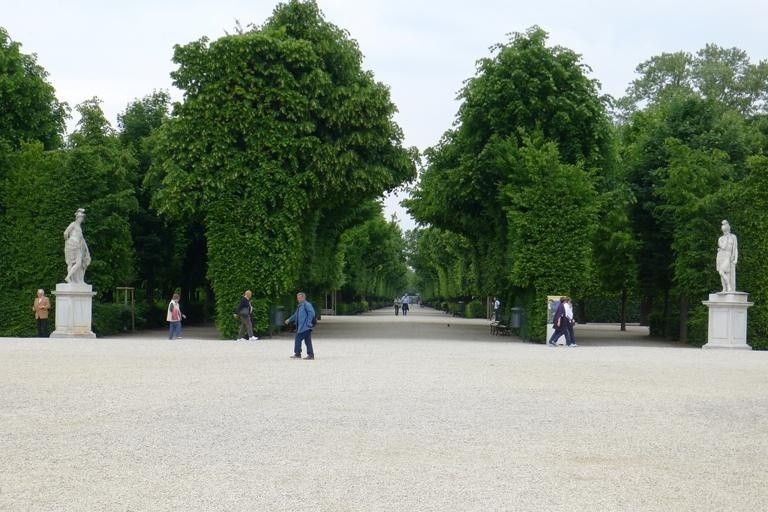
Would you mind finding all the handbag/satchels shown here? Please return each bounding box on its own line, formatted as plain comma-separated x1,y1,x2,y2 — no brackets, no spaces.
239,307,249,318
306,317,317,328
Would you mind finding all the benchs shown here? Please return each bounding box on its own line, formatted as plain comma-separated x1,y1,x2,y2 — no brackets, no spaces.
490,321,511,336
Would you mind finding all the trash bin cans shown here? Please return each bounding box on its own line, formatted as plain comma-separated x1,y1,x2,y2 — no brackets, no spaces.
273,305,285,335
457,301,464,318
510,307,525,342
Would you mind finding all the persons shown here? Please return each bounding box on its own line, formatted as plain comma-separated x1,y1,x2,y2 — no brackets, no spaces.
712,218,739,292
63,209,91,283
401,291,411,316
492,297,502,321
231,290,258,340
551,295,581,349
165,294,188,340
394,296,401,318
32,289,52,336
283,291,317,360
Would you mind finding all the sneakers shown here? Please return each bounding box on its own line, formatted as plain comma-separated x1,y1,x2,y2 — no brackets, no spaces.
566,342,578,346
237,336,258,341
303,356,314,359
548,340,557,347
289,354,301,358
169,337,182,339
